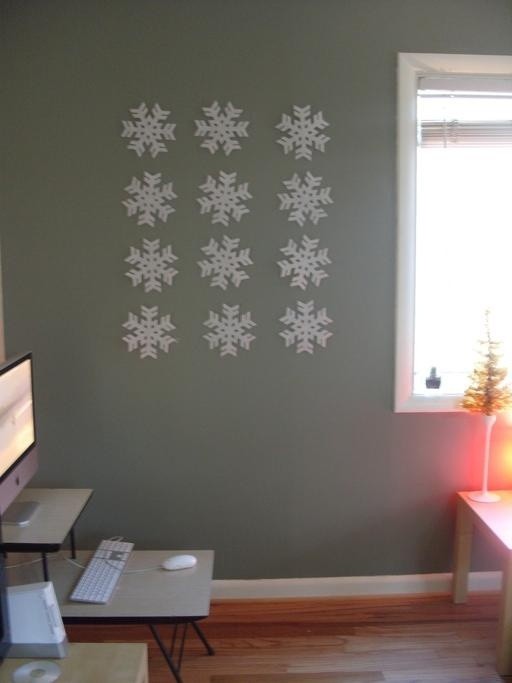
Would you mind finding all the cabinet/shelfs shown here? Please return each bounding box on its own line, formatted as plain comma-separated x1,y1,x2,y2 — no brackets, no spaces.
1,488,216,681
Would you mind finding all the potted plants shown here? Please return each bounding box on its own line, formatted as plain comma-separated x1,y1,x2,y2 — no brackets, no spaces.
460,329,511,504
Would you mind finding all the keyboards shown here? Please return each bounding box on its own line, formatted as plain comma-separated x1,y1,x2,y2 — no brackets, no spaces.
70,538,135,604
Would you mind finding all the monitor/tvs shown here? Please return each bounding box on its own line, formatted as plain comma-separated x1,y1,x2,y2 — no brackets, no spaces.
0,350,41,526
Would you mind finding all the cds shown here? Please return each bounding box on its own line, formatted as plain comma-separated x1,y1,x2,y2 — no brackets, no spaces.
10,660,62,683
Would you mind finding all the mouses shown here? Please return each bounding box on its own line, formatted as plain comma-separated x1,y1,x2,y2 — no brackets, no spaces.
160,554,197,571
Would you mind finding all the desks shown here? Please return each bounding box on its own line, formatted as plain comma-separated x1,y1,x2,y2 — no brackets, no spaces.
451,488,510,679
